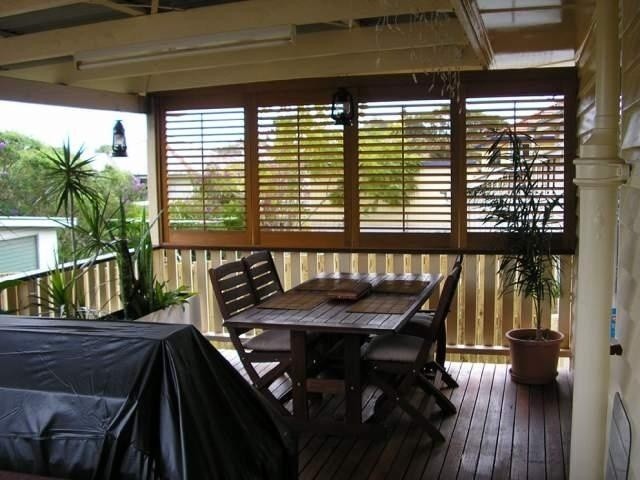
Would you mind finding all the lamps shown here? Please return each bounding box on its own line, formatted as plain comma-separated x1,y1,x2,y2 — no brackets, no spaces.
110,117,128,159
331,85,355,127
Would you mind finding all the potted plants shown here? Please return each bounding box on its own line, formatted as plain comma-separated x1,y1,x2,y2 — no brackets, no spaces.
30,143,201,335
474,131,565,388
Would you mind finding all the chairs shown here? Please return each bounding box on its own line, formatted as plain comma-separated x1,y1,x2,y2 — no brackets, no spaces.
402,250,459,390
206,260,321,421
242,249,345,383
361,267,458,443
